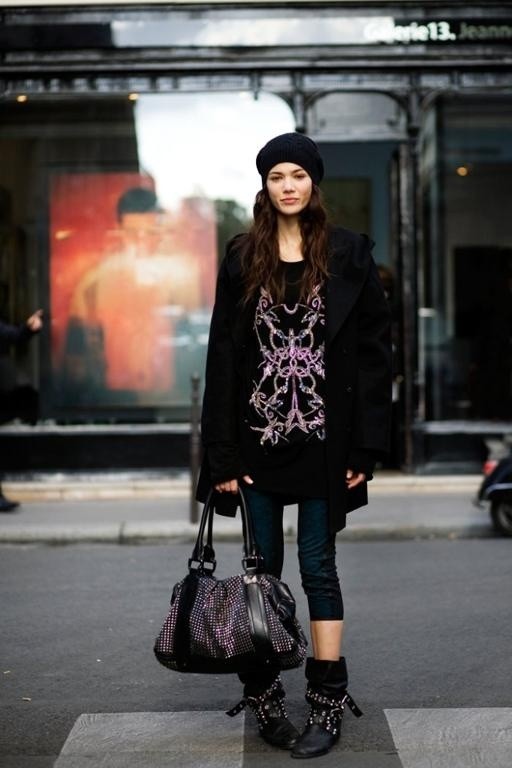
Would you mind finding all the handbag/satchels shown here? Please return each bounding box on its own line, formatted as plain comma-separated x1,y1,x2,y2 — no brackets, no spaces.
154,572,306,673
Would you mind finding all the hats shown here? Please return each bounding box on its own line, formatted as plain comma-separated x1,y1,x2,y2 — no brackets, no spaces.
257,132,324,184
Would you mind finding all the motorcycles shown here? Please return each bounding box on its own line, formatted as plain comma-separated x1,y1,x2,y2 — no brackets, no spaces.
475,439,512,537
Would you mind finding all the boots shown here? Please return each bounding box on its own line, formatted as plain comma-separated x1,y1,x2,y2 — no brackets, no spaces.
237,670,301,750
290,656,348,758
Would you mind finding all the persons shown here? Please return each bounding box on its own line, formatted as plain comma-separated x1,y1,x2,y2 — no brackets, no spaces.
195,132,392,759
68,187,219,402
0,308,45,511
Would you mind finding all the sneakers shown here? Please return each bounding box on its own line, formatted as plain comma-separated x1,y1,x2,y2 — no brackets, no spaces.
0,494,20,511
